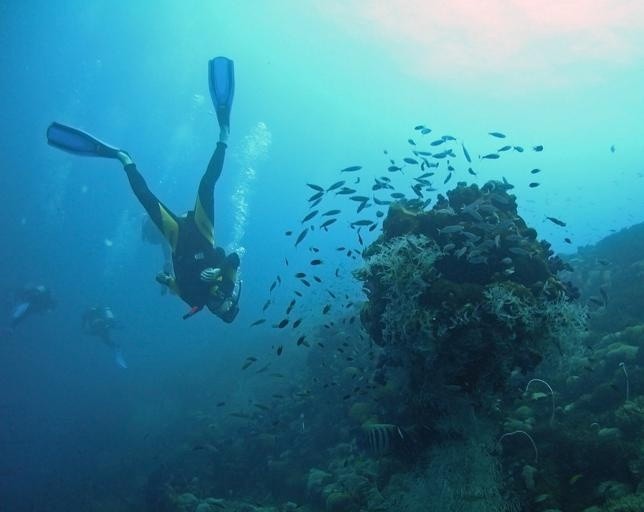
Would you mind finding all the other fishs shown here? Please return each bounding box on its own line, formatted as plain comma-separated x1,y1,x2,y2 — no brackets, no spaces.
217,121,615,511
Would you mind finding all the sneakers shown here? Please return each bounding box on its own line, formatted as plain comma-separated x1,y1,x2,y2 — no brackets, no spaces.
113,149,130,160
218,119,231,134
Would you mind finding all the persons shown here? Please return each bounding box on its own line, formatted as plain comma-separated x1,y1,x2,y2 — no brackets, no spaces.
46,56,242,324
